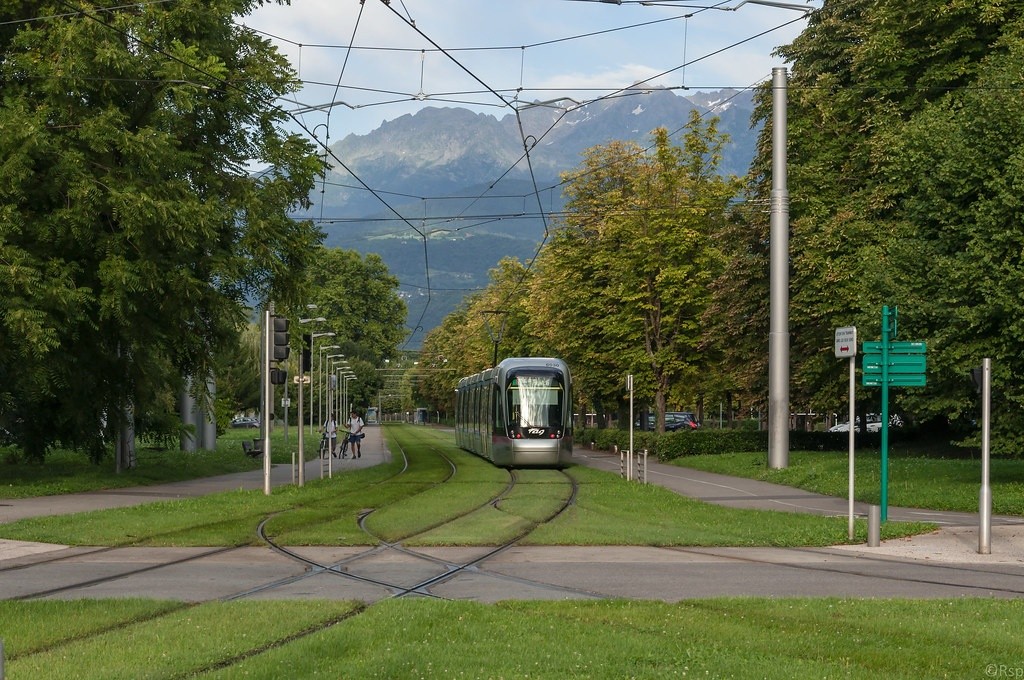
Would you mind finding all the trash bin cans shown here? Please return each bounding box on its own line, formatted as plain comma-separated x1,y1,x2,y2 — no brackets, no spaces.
253,438,265,459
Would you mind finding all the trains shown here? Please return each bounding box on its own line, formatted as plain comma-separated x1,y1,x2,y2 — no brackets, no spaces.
454,356,573,468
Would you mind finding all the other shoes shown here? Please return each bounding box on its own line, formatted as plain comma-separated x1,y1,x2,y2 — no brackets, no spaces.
351,456,356,459
358,451,361,458
332,451,337,458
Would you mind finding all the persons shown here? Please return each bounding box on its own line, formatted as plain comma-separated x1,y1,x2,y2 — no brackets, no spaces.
343,411,364,459
319,413,338,458
689,415,697,431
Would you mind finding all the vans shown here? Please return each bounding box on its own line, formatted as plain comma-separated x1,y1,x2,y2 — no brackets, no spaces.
828,413,902,434
635,412,701,431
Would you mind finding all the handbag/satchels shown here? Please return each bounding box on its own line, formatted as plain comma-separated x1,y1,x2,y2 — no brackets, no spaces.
360,433,365,438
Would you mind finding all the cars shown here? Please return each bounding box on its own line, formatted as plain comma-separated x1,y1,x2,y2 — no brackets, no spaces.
230,418,260,429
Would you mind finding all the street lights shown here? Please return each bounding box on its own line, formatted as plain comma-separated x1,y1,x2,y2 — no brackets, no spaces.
298,304,358,485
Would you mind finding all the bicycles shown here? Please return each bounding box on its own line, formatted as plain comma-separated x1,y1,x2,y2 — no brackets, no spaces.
338,429,355,459
315,431,330,458
